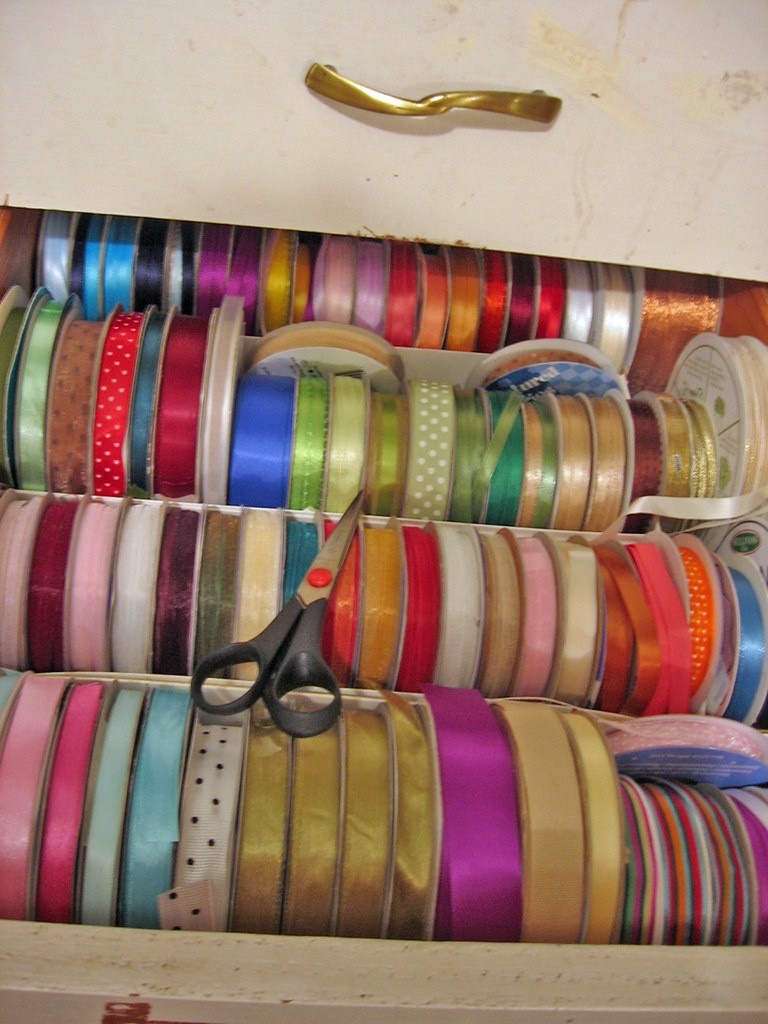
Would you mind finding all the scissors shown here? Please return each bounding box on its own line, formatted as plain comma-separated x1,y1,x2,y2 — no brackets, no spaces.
188,483,366,739
